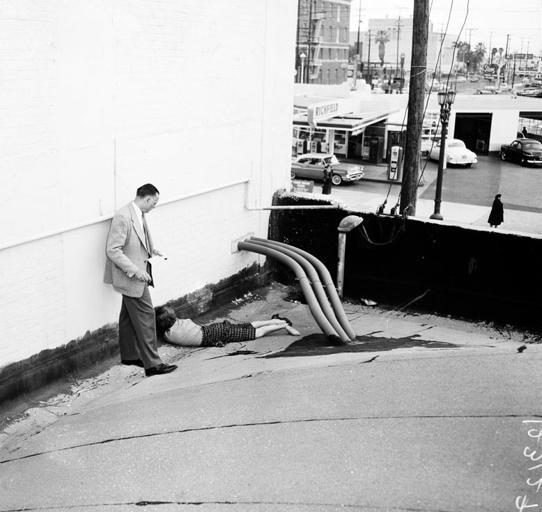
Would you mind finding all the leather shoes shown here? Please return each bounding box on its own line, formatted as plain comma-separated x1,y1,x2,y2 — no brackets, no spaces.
121,358,144,367
145,363,178,377
359,296,378,308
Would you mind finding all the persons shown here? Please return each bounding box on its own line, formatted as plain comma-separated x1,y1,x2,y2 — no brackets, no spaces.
321,165,332,195
488,194,504,228
103,184,178,375
156,306,300,347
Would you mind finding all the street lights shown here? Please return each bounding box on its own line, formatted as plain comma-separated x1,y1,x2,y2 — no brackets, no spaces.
299,52,306,84
398,52,406,94
428,88,456,221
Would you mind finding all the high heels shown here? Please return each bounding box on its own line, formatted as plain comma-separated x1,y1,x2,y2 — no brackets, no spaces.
272,314,292,326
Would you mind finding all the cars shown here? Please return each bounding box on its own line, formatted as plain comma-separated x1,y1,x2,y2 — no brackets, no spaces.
500,138,542,168
425,74,541,96
372,75,381,85
292,153,363,188
426,137,478,169
